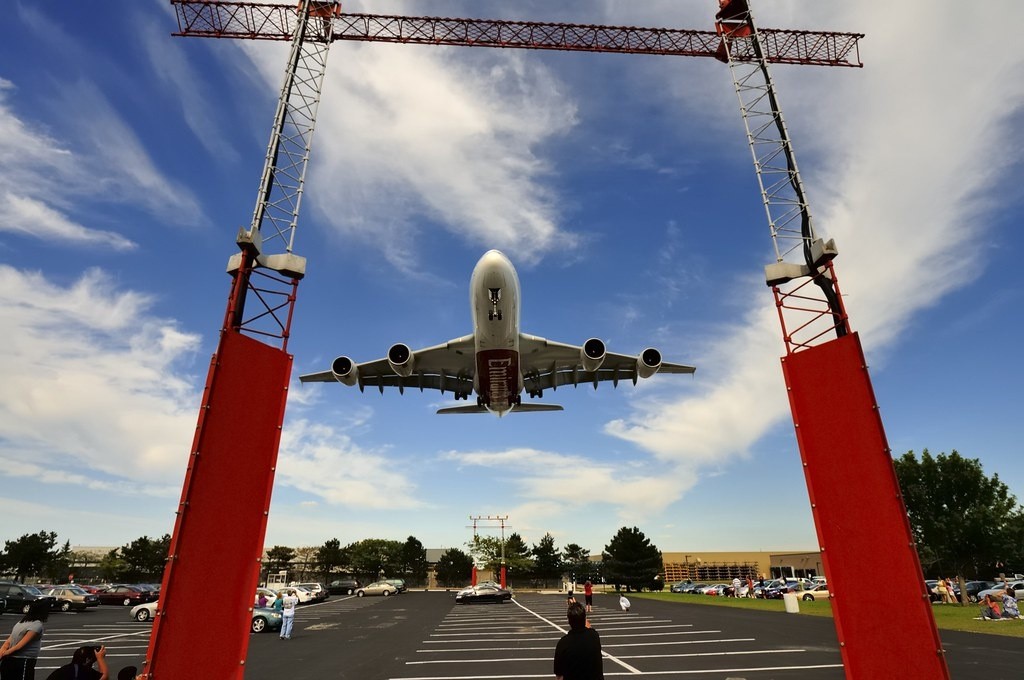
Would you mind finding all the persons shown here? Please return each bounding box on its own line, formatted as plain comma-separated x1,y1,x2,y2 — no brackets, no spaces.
554,602,604,680
565,578,577,606
924,575,959,604
747,576,757,599
758,576,767,599
619,593,631,610
46,644,137,680
797,578,804,591
686,578,691,584
0,598,49,680
732,576,742,598
972,581,1024,621
585,579,594,612
259,590,298,640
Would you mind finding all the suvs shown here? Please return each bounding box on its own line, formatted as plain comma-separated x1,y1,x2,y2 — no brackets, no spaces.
0,582,57,615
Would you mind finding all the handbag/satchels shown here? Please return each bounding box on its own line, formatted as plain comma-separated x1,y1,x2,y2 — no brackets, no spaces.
939,587,946,592
283,607,294,618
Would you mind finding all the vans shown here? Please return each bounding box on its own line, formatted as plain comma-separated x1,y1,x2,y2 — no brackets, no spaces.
374,578,407,594
323,579,358,596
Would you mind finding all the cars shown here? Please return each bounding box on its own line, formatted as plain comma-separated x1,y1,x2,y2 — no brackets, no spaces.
923,579,1004,603
976,580,1024,603
354,582,398,597
31,583,162,613
251,582,329,633
670,575,831,603
455,580,512,604
129,599,166,622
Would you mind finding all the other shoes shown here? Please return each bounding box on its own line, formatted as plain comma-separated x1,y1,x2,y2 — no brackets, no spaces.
983,616,991,620
586,610,593,613
943,601,947,603
279,637,284,640
751,596,756,599
764,598,767,600
286,636,291,639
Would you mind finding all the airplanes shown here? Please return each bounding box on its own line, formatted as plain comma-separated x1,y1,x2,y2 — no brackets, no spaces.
298,249,697,417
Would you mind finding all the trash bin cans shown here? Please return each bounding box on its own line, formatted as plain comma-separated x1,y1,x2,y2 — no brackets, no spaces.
783,594,800,613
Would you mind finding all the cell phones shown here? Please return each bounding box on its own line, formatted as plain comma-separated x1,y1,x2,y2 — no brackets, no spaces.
568,591,573,606
